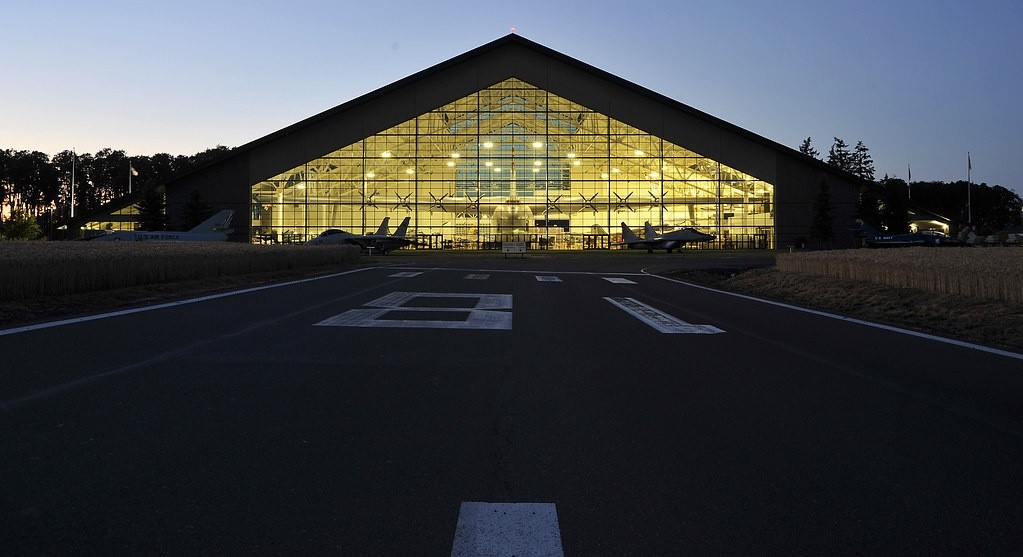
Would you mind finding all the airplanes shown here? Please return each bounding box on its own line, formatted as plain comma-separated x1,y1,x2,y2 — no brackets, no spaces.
610,220,716,254
304,215,411,255
73,209,236,241
